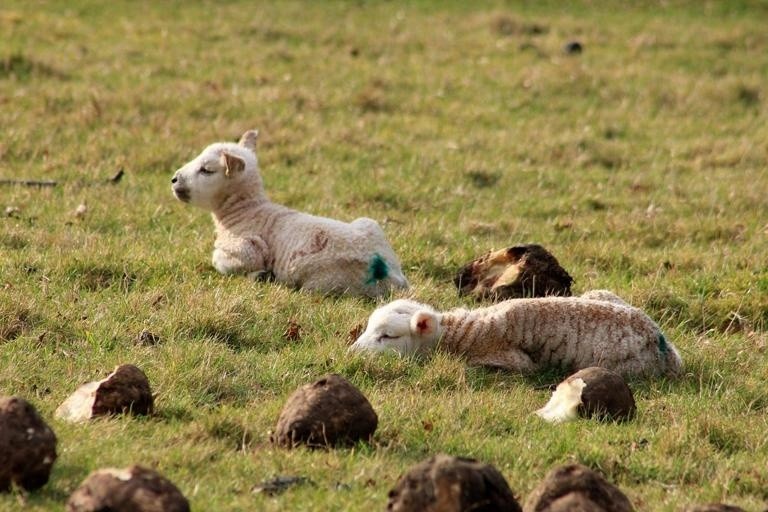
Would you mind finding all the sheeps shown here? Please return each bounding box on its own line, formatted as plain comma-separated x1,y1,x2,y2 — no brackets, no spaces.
172,130,408,304
346,290,685,384
